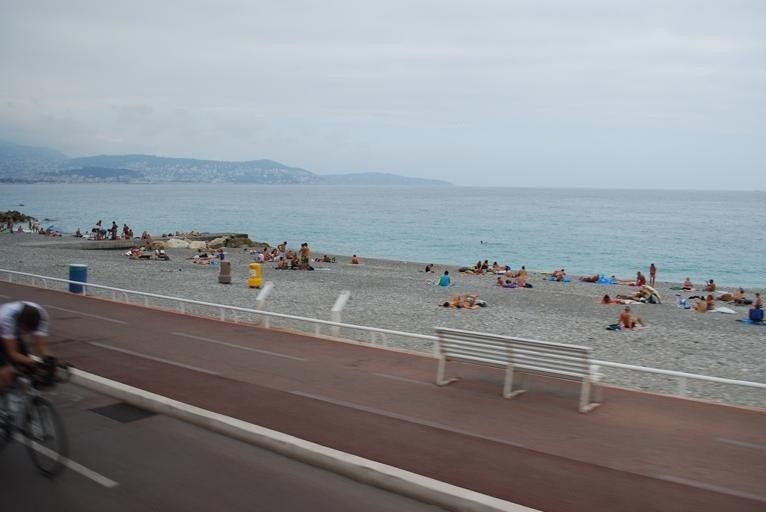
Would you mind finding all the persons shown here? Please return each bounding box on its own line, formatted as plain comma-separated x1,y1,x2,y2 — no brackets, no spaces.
1,214,62,237
459,259,527,288
175,229,224,265
75,219,133,240
0,299,61,424
438,270,451,286
424,263,434,272
128,230,169,261
578,263,656,329
255,241,314,272
438,291,488,309
350,254,359,264
323,255,330,262
681,277,765,325
552,269,566,282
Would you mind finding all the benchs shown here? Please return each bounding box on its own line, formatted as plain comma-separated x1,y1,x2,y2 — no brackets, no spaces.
431,324,605,413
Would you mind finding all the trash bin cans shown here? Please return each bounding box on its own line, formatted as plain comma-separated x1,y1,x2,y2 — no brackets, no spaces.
247,262,262,288
69,263,87,292
217,262,231,283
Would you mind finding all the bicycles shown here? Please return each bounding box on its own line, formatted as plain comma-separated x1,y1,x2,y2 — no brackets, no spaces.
1,360,76,478
